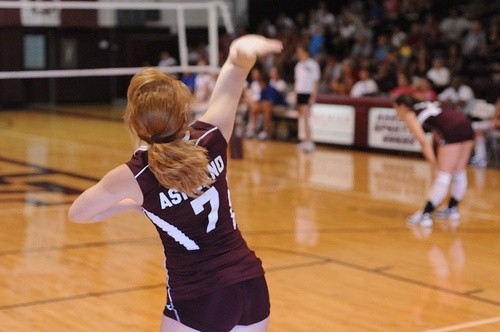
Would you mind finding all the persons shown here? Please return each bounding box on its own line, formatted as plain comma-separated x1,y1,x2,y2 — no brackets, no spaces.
257,0,500,167
68,34,284,332
155,51,286,140
393,92,474,226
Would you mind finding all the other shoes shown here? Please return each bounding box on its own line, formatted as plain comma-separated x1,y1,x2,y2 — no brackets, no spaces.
408,211,433,227
437,206,460,220
